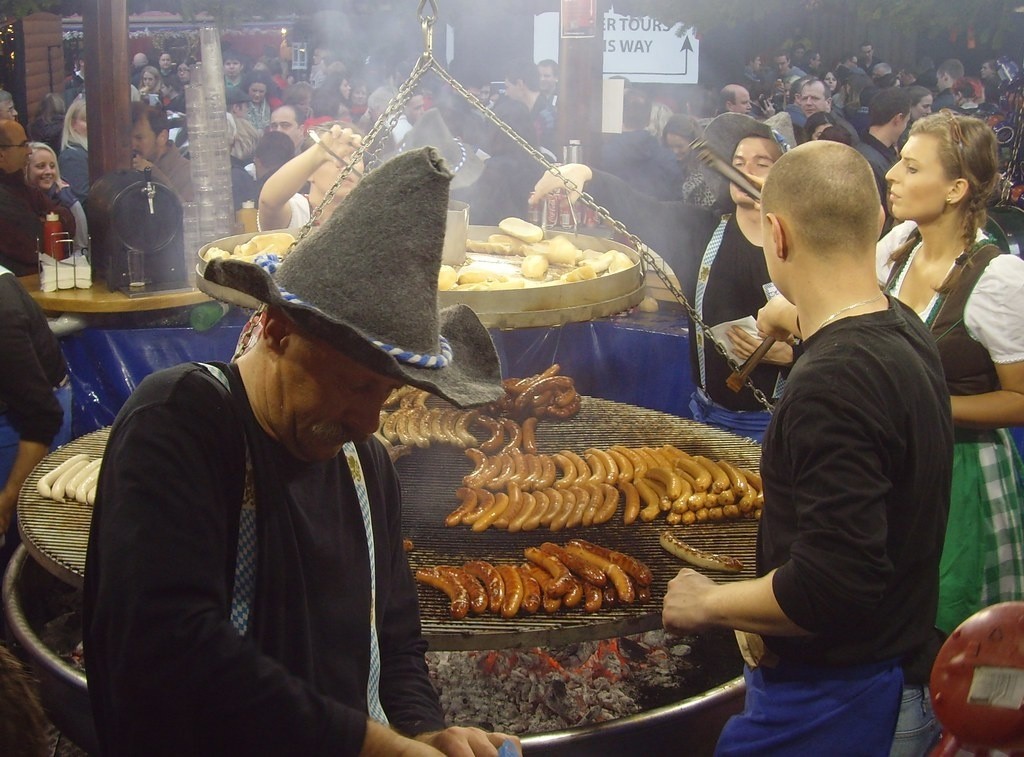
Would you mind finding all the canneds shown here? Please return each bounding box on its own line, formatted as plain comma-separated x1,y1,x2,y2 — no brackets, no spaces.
528,193,600,232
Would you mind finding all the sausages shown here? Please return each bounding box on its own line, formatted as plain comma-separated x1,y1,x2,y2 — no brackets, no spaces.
36,454,103,506
372,363,771,618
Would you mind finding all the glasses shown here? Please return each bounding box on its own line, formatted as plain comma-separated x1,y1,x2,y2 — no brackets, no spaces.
0,142,31,148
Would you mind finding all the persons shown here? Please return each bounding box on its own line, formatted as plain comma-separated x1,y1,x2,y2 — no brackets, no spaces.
0,261,70,631
874,111,1024,633
529,112,804,452
81,145,525,757
0,1,1023,309
662,140,953,756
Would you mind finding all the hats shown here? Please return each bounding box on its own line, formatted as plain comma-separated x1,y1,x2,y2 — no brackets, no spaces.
201,146,508,407
700,109,799,215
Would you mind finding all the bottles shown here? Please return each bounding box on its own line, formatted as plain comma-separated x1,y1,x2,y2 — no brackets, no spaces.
44,209,63,261
